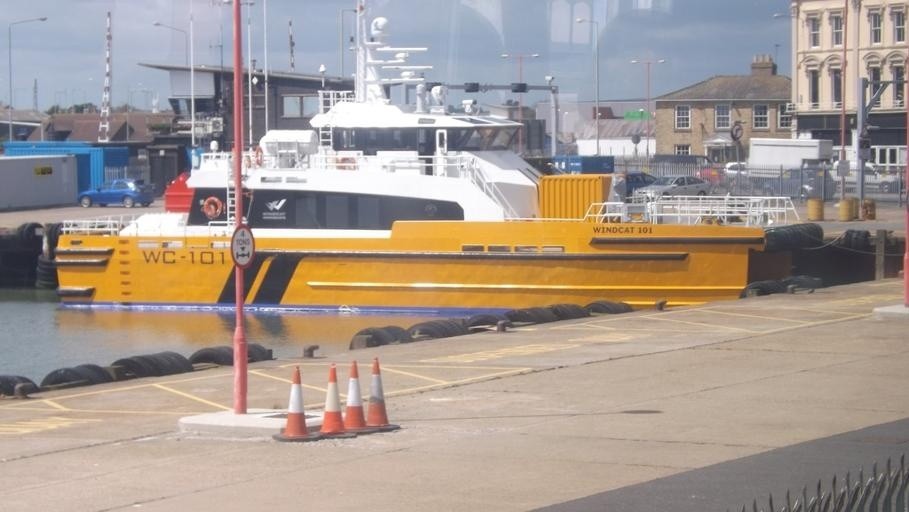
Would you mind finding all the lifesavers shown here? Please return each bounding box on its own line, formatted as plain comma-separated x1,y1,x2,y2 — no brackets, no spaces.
204,197,223,219
256,147,263,166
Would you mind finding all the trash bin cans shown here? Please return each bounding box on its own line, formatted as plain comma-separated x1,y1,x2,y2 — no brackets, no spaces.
861,198,876,220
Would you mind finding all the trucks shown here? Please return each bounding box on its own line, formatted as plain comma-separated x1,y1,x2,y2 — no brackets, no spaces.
746,137,837,196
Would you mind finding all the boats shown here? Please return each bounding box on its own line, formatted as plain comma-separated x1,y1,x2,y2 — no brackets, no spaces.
44,3,775,320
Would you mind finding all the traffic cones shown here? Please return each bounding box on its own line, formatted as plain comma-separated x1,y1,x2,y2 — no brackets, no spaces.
316,359,356,439
366,357,400,433
339,358,376,435
269,362,322,444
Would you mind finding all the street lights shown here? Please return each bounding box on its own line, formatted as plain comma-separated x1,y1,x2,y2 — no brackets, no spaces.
629,59,666,158
337,8,356,77
151,20,188,67
573,16,600,156
7,15,47,143
500,52,540,151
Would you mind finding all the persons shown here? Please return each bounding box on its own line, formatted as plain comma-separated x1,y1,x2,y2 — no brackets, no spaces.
255,142,263,169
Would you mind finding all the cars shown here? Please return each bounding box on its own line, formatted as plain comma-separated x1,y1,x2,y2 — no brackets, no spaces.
75,177,155,209
619,157,901,205
